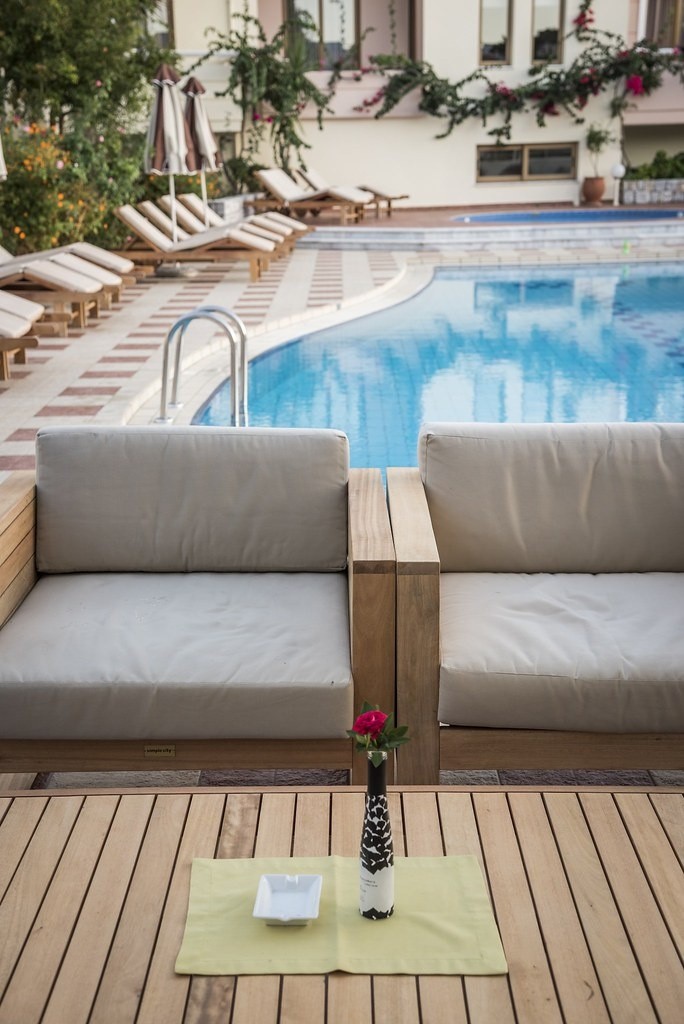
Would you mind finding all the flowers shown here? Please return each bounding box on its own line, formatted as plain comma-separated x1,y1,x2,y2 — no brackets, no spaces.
346,700,411,767
243,0,684,127
0,46,223,248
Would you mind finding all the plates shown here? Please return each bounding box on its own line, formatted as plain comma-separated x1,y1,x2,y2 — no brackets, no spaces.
252,874,323,926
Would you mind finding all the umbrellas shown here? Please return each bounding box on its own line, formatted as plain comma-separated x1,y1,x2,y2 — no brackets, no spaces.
144,64,220,243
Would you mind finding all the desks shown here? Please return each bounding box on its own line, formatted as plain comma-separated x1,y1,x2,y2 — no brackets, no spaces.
0,784,684,1024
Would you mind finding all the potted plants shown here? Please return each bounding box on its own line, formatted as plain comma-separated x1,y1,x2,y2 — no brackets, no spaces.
622,150,684,205
581,123,617,207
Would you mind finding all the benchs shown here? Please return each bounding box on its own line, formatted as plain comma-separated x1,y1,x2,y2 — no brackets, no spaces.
0,241,145,380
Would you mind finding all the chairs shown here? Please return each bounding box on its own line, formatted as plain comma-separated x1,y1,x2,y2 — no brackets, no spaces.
387,421,684,785
157,194,290,261
253,167,376,224
0,426,396,786
295,167,410,219
178,192,317,254
114,203,275,283
139,200,283,273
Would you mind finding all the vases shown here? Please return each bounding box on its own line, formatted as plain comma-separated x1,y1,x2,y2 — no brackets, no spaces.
357,751,395,920
